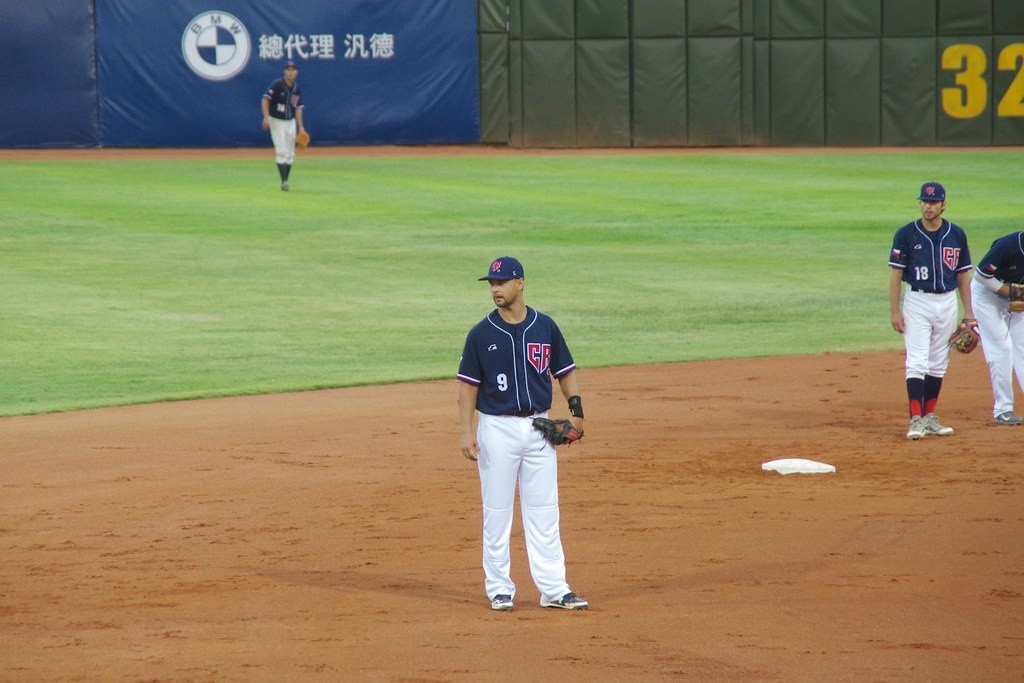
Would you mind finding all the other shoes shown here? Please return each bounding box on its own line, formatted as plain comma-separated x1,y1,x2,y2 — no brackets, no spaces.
281,181,288,190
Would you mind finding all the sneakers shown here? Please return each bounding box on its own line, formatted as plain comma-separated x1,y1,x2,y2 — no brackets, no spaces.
922,413,954,436
540,592,588,610
994,411,1023,424
906,415,925,440
491,595,513,610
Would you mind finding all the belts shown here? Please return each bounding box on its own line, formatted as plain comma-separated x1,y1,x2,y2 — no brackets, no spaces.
504,411,534,417
913,287,944,293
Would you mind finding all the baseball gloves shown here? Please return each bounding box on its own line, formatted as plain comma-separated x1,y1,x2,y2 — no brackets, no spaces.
295,131,310,148
532,417,583,445
1008,282,1024,313
948,319,981,354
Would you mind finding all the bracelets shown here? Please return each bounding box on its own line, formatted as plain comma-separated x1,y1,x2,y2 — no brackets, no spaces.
567,395,584,419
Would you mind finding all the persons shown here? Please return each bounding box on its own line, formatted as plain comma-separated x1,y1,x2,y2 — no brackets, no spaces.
888,182,980,440
261,61,310,192
457,256,589,611
969,229,1024,425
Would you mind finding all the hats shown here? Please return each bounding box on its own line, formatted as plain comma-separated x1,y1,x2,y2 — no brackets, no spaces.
283,60,297,70
478,256,524,280
915,182,945,200
